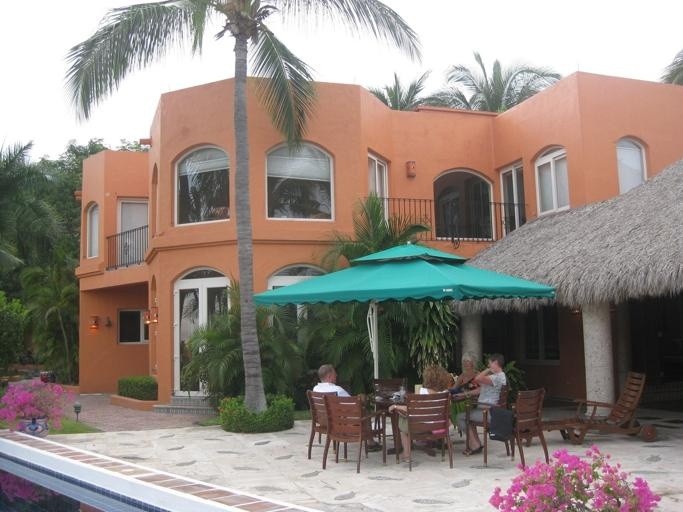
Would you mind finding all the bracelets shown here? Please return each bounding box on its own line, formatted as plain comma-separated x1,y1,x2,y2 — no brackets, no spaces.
394,404,397,409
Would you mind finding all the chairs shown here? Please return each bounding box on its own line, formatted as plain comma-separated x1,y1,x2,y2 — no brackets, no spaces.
371,376,403,444
304,388,339,461
320,393,388,473
463,382,511,456
543,371,651,444
395,390,455,473
482,385,551,471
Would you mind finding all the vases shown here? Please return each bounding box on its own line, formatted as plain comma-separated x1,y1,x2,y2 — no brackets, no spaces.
19,414,49,438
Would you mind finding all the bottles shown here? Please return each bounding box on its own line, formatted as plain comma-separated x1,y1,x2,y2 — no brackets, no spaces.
399,386,406,402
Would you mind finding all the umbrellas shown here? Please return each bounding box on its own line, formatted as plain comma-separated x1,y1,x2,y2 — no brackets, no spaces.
252,241,556,397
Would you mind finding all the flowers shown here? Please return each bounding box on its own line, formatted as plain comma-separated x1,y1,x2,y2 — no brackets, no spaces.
485,444,663,512
0,378,75,432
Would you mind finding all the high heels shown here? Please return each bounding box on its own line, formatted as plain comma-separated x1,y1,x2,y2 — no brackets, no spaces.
399,453,410,463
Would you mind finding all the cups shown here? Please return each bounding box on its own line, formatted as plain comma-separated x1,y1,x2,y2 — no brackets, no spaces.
414,384,423,394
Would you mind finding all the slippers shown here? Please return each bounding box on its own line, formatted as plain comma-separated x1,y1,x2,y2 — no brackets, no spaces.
463,443,484,456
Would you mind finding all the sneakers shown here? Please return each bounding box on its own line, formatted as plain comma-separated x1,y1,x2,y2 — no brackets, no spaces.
368,442,383,452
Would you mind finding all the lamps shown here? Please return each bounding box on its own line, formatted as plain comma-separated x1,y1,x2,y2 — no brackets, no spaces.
405,160,416,177
142,310,150,325
87,314,99,329
150,306,158,323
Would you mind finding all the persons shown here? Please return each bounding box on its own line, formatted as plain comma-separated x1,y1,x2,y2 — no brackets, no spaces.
456,353,507,456
450,352,480,437
313,364,382,452
388,365,452,462
